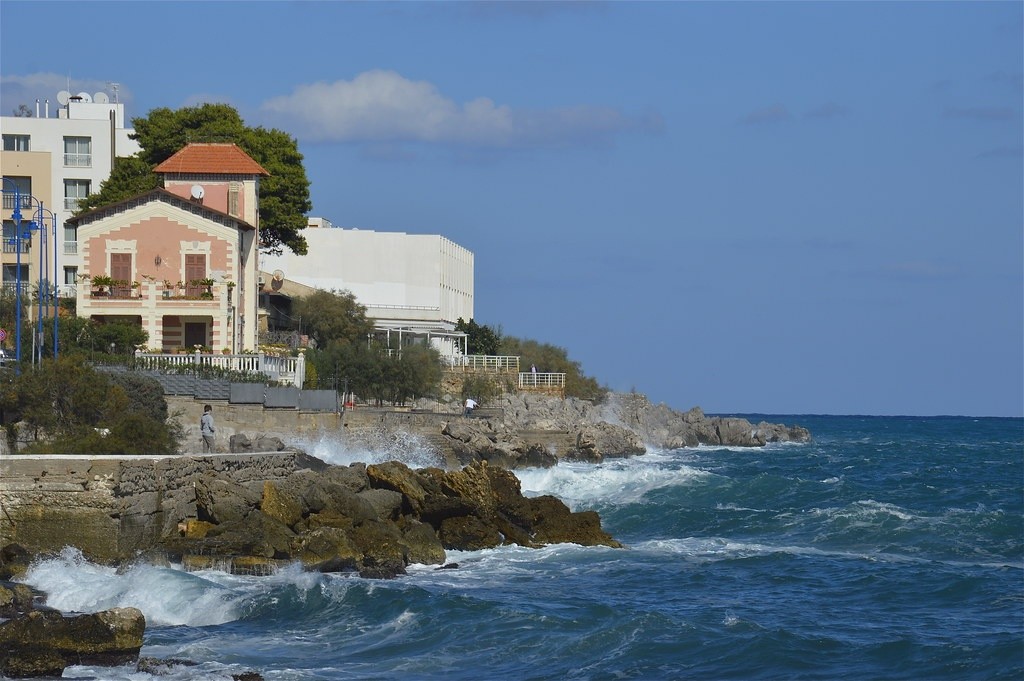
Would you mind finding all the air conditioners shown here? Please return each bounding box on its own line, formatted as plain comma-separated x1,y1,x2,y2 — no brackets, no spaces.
259,276,266,283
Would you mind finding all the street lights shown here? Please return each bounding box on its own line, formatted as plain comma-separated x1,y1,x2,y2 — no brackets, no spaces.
10,195,44,348
29,208,59,363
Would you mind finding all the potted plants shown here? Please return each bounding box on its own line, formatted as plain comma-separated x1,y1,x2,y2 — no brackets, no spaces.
222,348,231,355
161,346,211,355
227,282,236,291
91,275,214,289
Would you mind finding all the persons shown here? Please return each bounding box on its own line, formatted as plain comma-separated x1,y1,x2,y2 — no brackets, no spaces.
530,364,537,382
465,399,480,418
200,404,216,453
